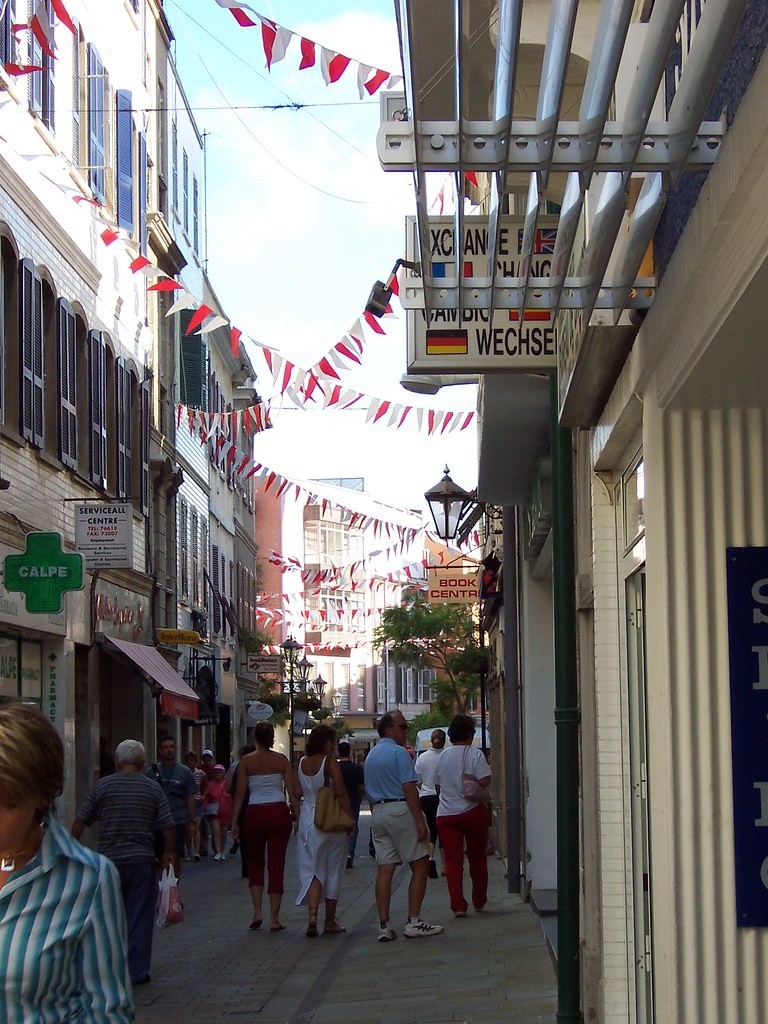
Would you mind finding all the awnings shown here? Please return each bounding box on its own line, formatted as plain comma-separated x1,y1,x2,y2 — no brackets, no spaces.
106,634,201,720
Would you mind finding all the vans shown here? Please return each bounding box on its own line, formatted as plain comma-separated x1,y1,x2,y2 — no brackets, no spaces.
413,725,491,794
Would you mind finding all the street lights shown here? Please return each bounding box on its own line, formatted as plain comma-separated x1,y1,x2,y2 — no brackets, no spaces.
279,634,304,773
331,689,344,718
297,650,314,738
311,673,328,724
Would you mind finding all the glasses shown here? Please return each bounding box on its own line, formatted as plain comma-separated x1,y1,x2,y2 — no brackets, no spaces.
399,723,408,729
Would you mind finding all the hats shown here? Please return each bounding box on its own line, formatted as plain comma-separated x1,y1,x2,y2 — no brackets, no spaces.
202,750,213,757
212,764,225,773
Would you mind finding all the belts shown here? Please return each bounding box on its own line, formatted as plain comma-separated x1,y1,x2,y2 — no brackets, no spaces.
373,798,406,804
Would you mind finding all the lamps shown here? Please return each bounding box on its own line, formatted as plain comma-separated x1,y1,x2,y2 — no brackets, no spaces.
365,259,421,318
423,463,502,540
399,373,480,395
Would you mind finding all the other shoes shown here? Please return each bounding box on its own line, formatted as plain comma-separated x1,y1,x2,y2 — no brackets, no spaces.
306,915,318,937
369,848,376,859
185,849,226,862
428,859,446,879
230,842,240,854
455,911,466,917
324,920,346,932
474,906,485,912
132,973,150,985
346,855,352,868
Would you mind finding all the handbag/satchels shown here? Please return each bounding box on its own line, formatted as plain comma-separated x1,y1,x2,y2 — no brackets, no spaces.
461,746,491,802
204,802,219,815
314,755,353,833
157,863,184,929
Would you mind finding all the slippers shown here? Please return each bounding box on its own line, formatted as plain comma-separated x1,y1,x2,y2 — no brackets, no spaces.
270,923,286,930
249,919,262,929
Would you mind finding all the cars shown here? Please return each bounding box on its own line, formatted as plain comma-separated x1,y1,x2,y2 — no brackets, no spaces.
473,719,491,731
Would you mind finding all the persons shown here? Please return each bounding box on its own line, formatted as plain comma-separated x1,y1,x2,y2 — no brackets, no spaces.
229,722,302,932
364,710,444,942
148,736,199,912
414,729,450,877
70,739,176,988
183,745,254,862
434,711,494,919
295,725,355,939
0,693,136,1024
334,741,365,870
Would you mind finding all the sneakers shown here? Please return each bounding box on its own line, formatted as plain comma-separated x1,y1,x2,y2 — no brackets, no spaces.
378,926,396,942
404,921,443,938
